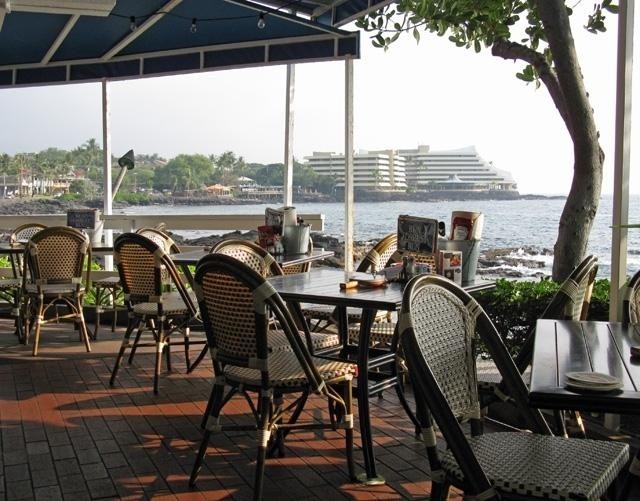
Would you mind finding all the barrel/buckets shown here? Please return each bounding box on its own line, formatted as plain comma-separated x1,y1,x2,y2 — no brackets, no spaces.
438,239,482,281
89,221,104,242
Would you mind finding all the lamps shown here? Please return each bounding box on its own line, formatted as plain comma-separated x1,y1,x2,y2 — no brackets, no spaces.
109,4,290,35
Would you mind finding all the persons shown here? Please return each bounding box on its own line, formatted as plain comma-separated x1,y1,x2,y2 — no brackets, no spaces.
451,255,459,265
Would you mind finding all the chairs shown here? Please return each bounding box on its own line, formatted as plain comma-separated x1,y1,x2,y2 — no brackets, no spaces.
1,214,94,359
403,272,632,499
463,252,599,442
92,222,167,343
623,267,640,323
180,240,359,500
105,227,208,399
301,230,426,433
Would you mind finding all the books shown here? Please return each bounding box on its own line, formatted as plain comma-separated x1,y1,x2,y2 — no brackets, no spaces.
398,213,439,253
449,210,483,240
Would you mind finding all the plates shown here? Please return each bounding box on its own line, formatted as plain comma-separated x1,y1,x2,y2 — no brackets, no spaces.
563,370,624,395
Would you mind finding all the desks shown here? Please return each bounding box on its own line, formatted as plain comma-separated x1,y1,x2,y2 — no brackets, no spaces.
526,317,640,413
162,247,336,375
1,242,114,278
260,263,497,434
